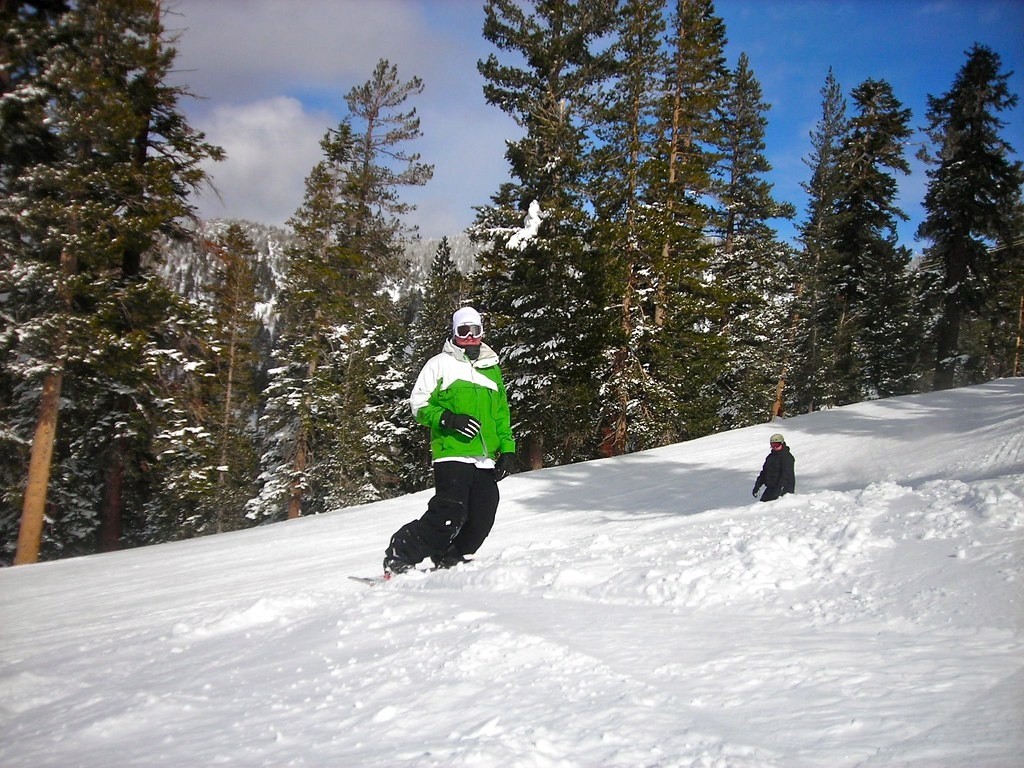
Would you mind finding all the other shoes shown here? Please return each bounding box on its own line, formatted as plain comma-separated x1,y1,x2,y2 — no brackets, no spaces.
436,556,473,570
384,558,416,580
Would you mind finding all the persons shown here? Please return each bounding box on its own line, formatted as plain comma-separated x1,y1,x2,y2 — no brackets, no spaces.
751,434,796,504
384,306,519,575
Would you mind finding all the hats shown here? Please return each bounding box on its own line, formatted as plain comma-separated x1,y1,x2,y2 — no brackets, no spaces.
452,307,482,327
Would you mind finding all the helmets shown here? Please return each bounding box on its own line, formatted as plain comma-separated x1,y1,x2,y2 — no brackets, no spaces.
770,434,784,443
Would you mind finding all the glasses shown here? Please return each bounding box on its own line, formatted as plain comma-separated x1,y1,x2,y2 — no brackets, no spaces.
454,324,483,339
770,442,783,447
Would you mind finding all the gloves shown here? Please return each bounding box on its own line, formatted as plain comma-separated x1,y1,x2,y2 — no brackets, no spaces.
440,408,482,440
492,452,518,483
